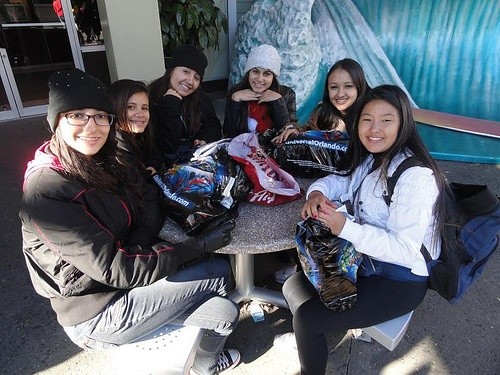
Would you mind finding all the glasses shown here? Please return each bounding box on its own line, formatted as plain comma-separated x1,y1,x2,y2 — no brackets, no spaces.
62,112,115,126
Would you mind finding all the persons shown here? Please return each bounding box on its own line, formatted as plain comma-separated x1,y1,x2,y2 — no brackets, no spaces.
223,44,298,138
147,46,222,165
92,80,174,248
271,58,372,284
281,85,470,375
19,67,241,375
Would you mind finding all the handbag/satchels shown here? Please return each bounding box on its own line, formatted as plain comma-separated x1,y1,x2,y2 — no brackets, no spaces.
273,128,359,179
228,132,311,203
193,139,255,199
382,156,500,302
296,213,363,313
153,198,240,237
153,164,235,211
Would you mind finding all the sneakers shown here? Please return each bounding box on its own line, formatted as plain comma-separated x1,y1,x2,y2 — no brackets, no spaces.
212,348,241,373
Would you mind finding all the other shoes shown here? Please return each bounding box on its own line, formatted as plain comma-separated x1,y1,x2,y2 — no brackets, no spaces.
272,265,297,283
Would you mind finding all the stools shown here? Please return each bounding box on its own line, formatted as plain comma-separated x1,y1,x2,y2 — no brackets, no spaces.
100,325,205,375
361,311,414,351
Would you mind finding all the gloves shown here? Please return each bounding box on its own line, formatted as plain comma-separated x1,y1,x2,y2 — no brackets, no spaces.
174,221,236,265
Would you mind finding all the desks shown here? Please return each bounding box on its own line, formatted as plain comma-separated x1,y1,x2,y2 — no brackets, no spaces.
159,178,315,307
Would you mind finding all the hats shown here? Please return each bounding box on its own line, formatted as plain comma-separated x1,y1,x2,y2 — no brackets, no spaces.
46,69,114,133
166,44,209,81
244,44,281,76
